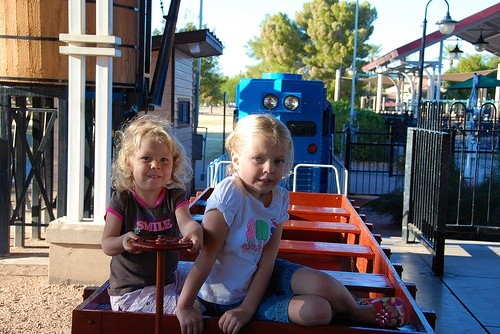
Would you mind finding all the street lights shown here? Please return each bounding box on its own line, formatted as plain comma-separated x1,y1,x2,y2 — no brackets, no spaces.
416,0,460,128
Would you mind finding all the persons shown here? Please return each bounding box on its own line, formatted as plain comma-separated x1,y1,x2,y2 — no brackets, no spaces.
175,115,408,334
101,114,204,315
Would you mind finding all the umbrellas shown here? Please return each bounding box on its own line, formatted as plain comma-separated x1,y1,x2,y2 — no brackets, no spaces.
448,74,500,89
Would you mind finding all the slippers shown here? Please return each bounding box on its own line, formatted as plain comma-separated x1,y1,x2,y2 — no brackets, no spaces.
355,296,409,328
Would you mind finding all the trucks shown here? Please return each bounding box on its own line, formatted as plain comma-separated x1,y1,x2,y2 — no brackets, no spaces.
70,72,438,334
